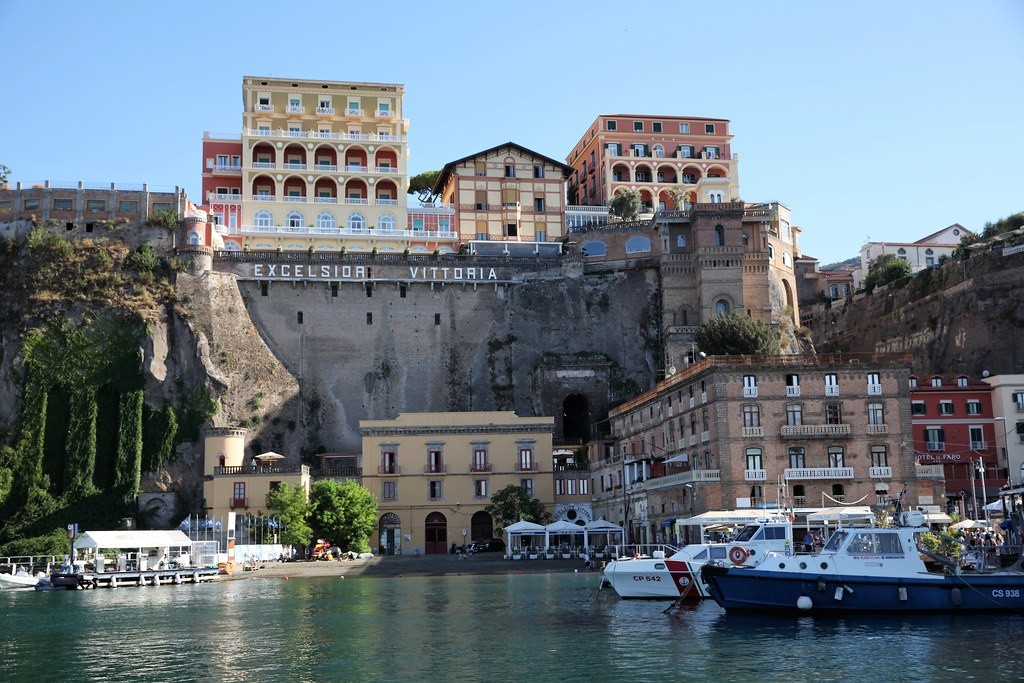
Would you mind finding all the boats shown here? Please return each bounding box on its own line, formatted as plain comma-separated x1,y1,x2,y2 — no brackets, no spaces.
604,484,1024,619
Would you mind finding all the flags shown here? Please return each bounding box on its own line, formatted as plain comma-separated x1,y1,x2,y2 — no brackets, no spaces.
267,518,284,528
178,516,222,530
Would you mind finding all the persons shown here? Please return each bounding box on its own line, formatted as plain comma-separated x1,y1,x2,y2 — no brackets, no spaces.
251,459,257,473
803,531,813,553
956,527,1003,552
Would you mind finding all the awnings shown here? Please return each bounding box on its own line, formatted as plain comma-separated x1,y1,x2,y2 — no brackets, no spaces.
923,513,953,524
661,518,676,527
661,454,688,463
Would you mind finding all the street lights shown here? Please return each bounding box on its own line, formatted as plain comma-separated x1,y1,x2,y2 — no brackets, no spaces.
993,417,1012,489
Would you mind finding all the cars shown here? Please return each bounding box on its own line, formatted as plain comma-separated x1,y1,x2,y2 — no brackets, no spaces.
477,538,505,552
975,520,992,531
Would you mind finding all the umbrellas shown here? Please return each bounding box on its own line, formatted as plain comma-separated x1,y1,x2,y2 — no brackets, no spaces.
702,524,733,540
255,451,285,467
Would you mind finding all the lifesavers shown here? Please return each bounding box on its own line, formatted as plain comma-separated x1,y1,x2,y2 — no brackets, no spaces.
790,514,795,523
729,547,747,564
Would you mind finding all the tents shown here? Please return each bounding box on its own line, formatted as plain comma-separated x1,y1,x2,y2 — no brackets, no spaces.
502,517,624,559
676,511,788,550
806,507,877,553
950,519,985,536
982,497,1015,519
